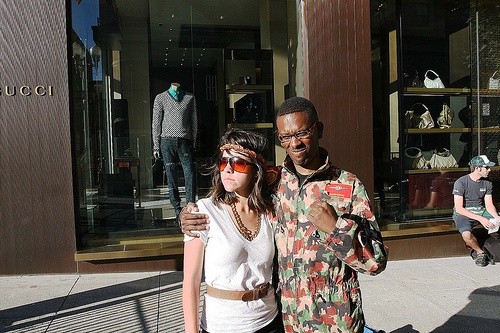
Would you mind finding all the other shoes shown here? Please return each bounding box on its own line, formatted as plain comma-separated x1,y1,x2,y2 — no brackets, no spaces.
475,253,487,266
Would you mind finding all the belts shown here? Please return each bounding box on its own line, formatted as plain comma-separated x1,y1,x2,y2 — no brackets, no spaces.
204,283,274,302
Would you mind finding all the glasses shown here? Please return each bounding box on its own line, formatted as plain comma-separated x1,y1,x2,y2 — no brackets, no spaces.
218,156,257,174
278,120,317,143
485,166,490,170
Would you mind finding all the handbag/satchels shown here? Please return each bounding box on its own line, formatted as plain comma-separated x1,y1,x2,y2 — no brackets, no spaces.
459,96,474,128
424,70,445,89
409,103,434,129
487,70,500,90
437,105,454,129
429,148,459,168
406,72,423,87
404,147,429,170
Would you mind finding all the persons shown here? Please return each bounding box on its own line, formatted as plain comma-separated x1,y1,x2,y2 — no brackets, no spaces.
152,83,197,221
452,155,500,266
411,174,449,209
183,128,285,333
178,96,389,333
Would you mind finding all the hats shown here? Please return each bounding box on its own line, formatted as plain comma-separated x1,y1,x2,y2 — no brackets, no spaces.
471,155,495,166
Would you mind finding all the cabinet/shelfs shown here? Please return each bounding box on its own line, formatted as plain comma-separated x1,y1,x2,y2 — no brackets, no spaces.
225,49,275,166
403,87,500,220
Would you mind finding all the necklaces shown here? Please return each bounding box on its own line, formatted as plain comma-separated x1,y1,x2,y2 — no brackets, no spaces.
229,200,261,241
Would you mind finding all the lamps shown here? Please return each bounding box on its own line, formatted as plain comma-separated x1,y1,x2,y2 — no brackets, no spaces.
73,41,101,72
